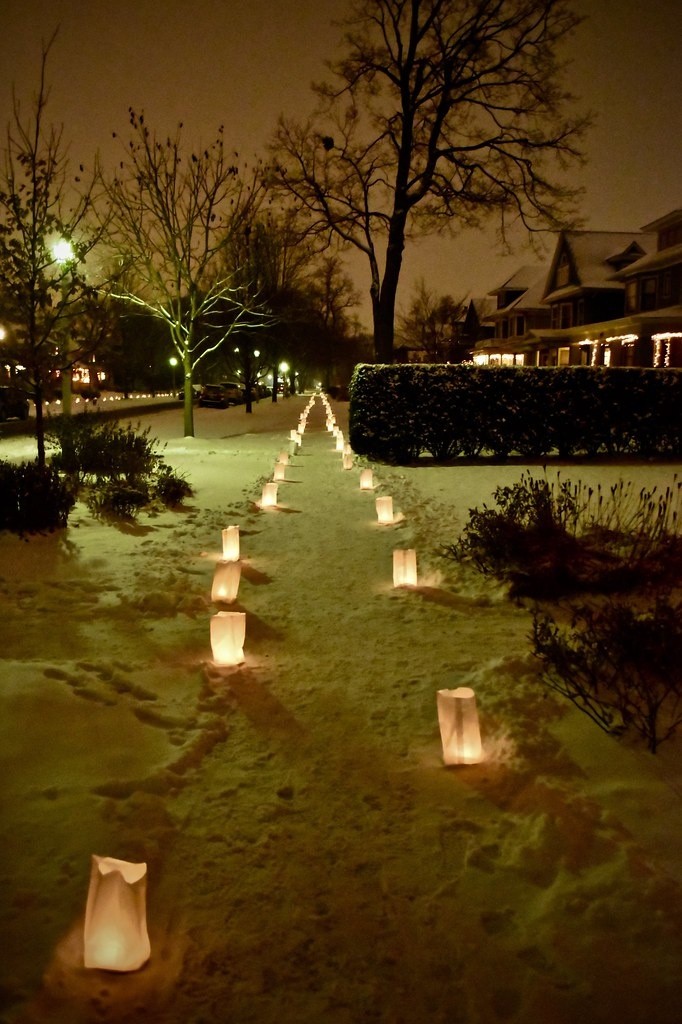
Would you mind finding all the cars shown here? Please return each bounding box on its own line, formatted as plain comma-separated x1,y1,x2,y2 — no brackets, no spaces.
178,384,204,400
242,379,287,401
0,355,110,426
198,383,229,410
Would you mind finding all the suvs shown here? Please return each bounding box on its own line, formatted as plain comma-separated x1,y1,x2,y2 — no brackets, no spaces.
217,381,246,405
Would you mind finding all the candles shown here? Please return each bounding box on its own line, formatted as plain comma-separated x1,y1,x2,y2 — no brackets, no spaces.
81,388,482,974
